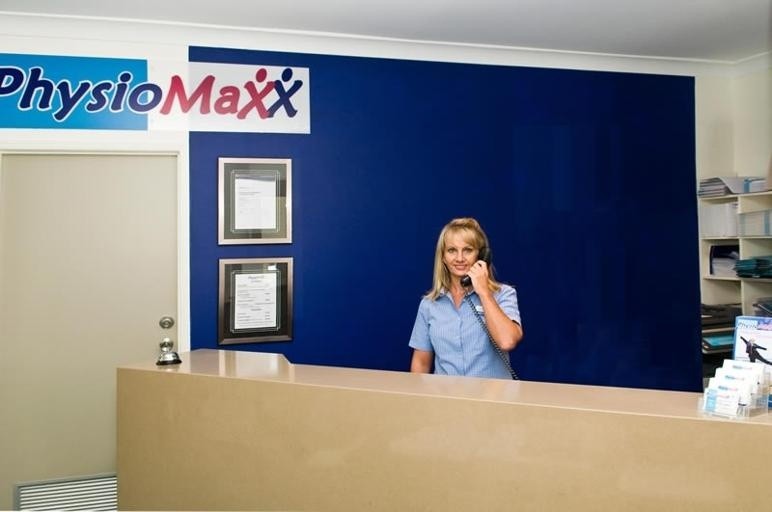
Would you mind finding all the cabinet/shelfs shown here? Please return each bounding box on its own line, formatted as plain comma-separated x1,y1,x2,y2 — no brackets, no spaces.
698,191,772,315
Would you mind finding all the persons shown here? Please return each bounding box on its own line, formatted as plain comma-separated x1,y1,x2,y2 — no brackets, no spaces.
408,216,525,380
738,335,772,366
696,173,772,328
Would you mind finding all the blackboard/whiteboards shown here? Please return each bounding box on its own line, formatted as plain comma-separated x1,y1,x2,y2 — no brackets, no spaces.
460,248,492,287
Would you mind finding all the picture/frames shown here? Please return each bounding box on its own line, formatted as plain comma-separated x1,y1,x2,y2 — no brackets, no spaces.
218,157,293,245
218,256,293,346
